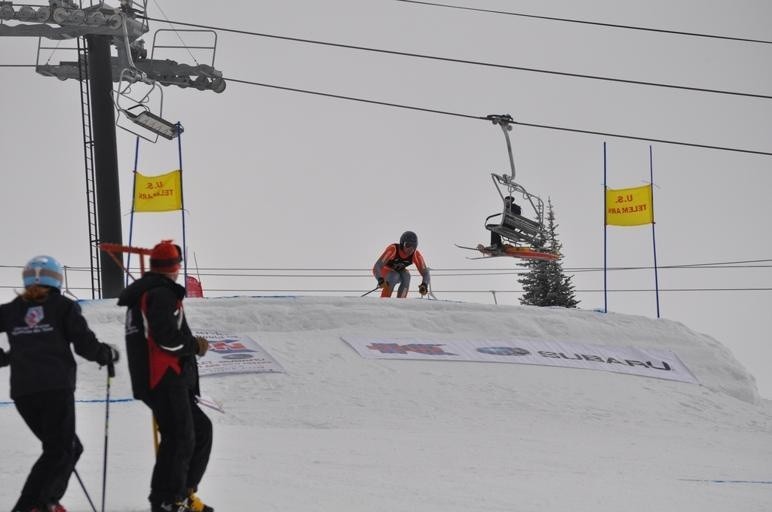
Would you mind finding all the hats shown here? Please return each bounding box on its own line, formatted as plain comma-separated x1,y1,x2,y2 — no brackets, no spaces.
149,239,182,273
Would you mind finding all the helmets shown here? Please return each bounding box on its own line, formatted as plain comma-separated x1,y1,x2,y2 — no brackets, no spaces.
22,254,64,293
400,230,417,254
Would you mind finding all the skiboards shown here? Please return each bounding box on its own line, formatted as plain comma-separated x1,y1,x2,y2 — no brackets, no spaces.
453,243,560,259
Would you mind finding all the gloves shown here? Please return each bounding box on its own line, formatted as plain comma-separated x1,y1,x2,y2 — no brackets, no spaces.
419,282,428,295
99,345,116,367
0,347,11,369
193,335,207,356
377,277,387,288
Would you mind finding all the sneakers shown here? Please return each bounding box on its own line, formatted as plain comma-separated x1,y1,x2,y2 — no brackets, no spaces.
183,489,213,512
151,498,192,512
21,498,67,512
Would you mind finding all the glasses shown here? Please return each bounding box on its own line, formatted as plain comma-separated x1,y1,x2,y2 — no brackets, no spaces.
403,241,417,249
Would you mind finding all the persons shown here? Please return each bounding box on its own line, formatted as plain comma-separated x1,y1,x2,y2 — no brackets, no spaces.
117,240,213,512
373,231,430,298
486,197,521,251
0,256,119,512
